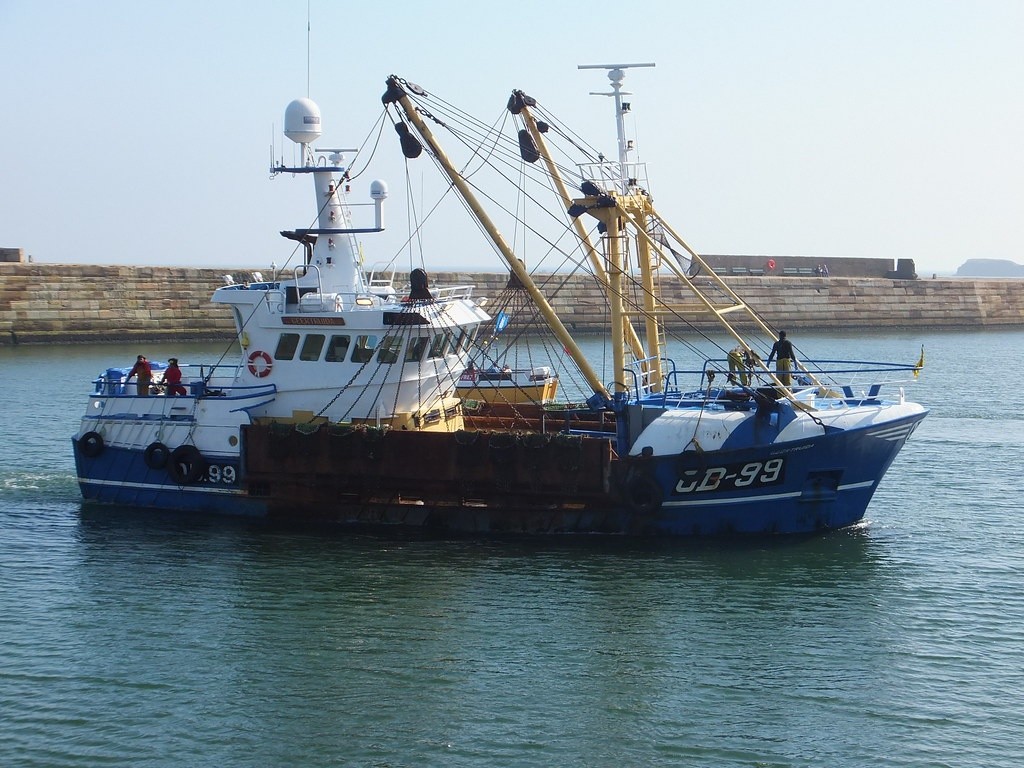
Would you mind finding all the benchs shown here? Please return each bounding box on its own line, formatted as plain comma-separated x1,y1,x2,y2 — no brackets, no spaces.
783,267,798,275
798,267,812,276
732,268,745,275
750,269,763,275
711,267,727,275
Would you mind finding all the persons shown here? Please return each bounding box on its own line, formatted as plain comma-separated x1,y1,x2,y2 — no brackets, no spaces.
766,331,798,398
817,264,828,277
158,358,186,395
502,365,512,373
727,344,749,386
486,361,499,373
124,354,154,395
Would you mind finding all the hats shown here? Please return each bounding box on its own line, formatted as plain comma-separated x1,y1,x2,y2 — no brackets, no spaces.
779,331,787,337
137,354,146,359
168,358,178,364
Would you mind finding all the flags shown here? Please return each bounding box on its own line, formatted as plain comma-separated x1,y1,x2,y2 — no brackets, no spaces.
912,349,923,376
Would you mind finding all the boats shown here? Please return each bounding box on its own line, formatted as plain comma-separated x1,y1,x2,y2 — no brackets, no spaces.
70,63,934,538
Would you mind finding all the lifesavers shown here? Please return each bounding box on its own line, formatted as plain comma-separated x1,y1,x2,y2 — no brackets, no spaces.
768,260,775,269
144,443,203,483
248,350,273,378
78,431,103,457
